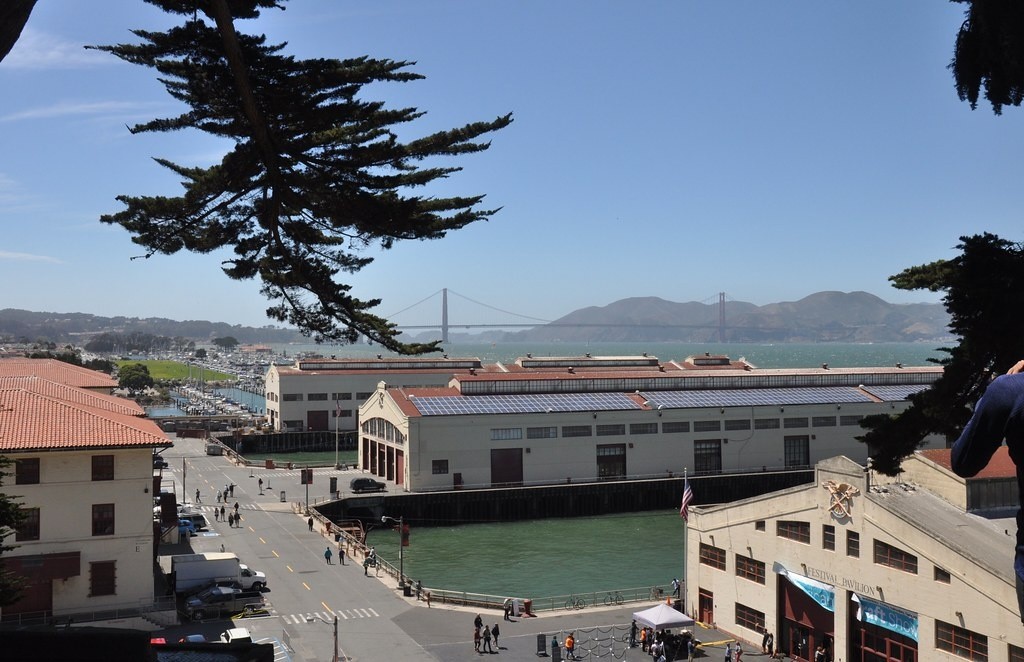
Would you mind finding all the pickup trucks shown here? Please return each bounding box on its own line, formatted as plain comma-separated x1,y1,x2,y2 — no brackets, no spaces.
182,584,265,622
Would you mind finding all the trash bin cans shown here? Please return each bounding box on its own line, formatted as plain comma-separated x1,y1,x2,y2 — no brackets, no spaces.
335,535,340,542
404,586,411,596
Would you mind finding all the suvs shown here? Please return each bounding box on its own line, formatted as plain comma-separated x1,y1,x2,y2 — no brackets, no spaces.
349,477,385,493
177,512,206,532
186,578,249,600
220,627,253,644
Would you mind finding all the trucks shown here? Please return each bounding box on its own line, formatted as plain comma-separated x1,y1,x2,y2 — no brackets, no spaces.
171,551,267,595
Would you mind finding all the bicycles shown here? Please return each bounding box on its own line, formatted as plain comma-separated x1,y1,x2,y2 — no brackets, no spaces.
603,591,624,606
564,592,586,611
649,584,660,601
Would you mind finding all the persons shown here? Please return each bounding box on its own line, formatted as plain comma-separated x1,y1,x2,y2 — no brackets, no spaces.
339,547,346,565
324,546,332,565
949,359,1024,626
219,544,225,553
363,547,376,575
815,647,831,662
566,632,576,660
630,619,698,662
473,614,500,655
504,598,512,620
415,579,423,599
196,488,201,503
671,578,681,598
259,478,263,494
308,516,313,531
551,636,558,648
214,501,242,528
216,483,237,502
725,629,773,662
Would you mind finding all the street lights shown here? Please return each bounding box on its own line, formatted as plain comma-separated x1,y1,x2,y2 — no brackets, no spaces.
382,515,408,591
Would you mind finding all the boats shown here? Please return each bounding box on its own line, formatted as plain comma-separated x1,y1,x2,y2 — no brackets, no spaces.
336,518,367,546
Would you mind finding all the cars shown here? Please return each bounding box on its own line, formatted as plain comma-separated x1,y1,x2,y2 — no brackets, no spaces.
159,519,196,537
152,454,169,469
153,502,191,519
183,634,205,642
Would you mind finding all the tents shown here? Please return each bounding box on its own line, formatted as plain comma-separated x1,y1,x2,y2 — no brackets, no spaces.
632,602,695,636
